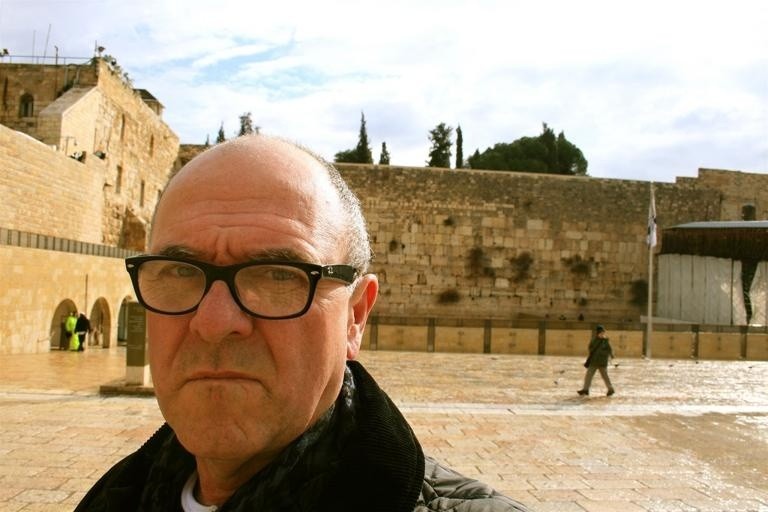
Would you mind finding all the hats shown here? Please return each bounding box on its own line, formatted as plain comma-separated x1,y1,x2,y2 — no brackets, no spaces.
597,326,605,334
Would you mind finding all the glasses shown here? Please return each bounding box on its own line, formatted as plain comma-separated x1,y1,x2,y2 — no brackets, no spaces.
124,254,358,319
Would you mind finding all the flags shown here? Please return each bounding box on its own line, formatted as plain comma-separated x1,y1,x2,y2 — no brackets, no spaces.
646,185,659,248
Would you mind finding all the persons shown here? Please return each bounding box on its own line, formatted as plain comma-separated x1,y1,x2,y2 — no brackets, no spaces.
578,323,615,398
74,311,92,351
59,313,69,350
72,131,539,512
65,312,81,351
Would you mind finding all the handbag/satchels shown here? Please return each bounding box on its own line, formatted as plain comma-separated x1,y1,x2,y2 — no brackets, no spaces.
584,359,590,368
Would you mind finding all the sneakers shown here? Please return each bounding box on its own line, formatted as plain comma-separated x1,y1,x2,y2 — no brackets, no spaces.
577,390,588,395
607,389,614,396
